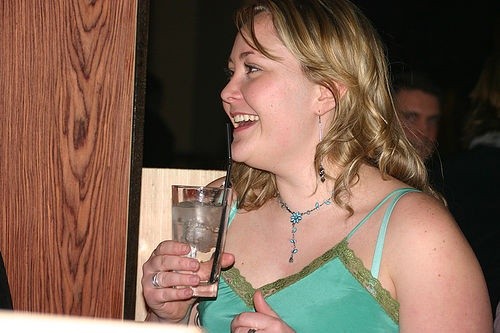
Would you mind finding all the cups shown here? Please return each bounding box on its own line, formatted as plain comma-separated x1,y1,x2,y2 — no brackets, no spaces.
172,185,233,299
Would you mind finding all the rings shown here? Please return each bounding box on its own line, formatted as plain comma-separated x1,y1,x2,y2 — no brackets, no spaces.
248,328,256,333
151,272,160,287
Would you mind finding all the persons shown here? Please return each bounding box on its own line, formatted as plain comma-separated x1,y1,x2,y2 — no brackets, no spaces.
440,66,500,320
141,0,494,333
389,67,438,164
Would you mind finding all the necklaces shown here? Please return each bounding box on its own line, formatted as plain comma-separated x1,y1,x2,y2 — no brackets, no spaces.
276,192,334,264
